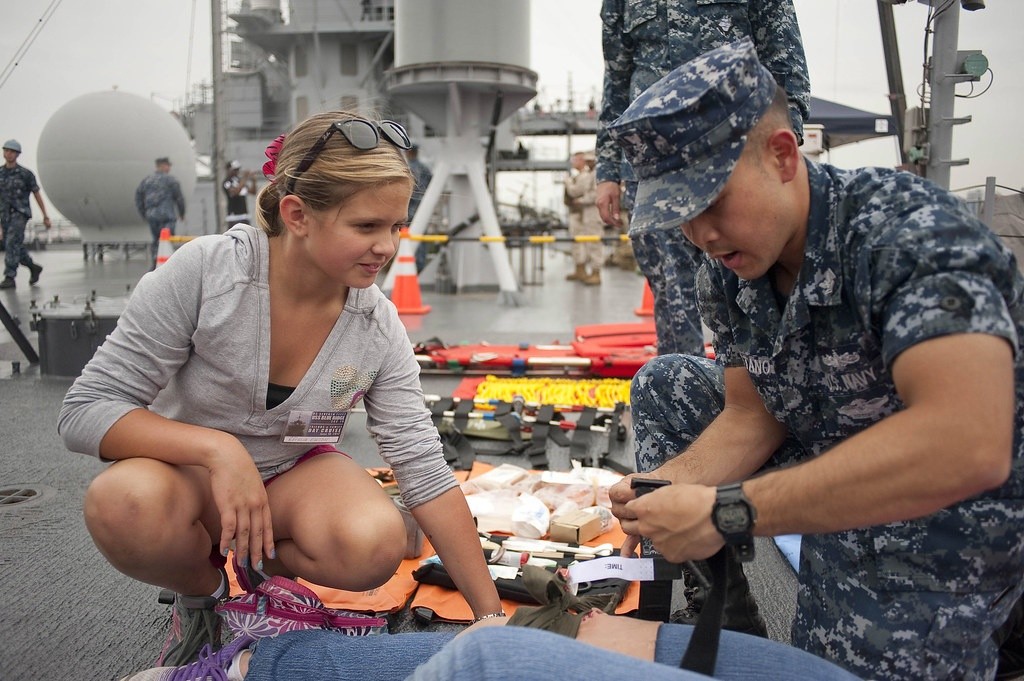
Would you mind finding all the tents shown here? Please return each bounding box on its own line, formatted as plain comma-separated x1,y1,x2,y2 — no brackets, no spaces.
805,97,900,165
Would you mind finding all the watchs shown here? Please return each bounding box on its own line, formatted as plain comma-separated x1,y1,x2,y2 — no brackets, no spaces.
711,482,758,562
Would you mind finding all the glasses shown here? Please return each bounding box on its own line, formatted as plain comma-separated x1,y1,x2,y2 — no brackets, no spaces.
285,118,413,196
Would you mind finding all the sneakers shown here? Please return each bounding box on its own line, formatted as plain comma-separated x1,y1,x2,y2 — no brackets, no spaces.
120,631,255,681
156,568,230,667
232,548,296,594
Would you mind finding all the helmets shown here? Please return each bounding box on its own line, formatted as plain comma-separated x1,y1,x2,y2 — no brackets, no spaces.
2,139,22,153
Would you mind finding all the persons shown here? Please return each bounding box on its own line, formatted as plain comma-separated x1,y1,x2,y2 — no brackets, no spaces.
405,142,432,274
361,0,373,21
564,152,605,286
0,139,51,288
222,159,257,230
594,0,811,357
121,607,864,680
58,110,501,668
135,157,185,270
606,35,1024,681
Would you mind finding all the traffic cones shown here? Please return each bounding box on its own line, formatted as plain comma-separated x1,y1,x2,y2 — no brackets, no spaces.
391,227,432,315
155,228,176,269
634,278,656,316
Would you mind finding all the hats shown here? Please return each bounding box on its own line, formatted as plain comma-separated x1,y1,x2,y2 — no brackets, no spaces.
605,35,780,240
155,156,172,165
226,160,242,170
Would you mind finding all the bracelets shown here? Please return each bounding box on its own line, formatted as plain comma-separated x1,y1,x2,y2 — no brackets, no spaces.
44,218,49,221
472,609,506,624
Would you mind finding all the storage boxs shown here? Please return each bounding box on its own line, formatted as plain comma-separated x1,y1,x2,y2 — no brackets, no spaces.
550,510,603,546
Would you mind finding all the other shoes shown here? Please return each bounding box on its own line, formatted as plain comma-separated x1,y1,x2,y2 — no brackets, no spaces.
668,586,769,638
582,268,601,285
0,275,16,288
29,264,43,284
566,263,589,281
